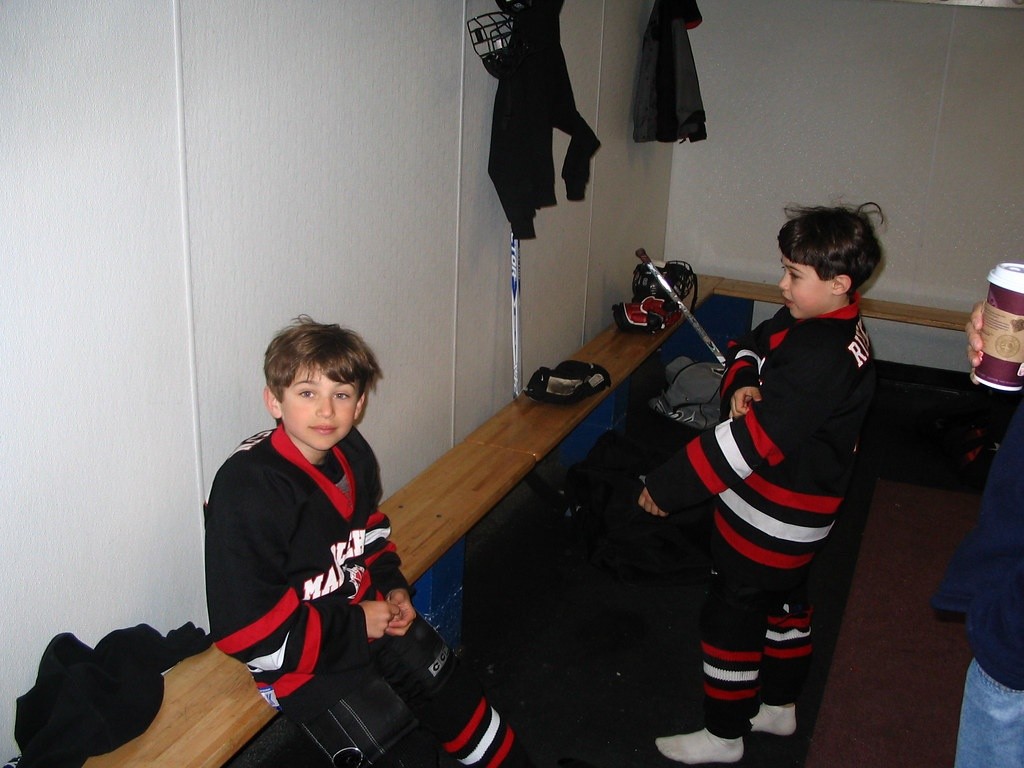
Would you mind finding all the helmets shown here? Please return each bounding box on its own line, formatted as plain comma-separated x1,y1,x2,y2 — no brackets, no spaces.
632,260,694,301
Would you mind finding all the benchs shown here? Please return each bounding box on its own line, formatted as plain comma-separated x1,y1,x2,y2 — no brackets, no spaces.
81,274,971,768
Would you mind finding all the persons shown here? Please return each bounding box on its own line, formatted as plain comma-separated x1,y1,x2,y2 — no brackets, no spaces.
930,291,1024,767
637,202,883,765
203,322,535,768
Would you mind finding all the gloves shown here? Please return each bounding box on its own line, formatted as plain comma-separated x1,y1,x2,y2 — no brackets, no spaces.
612,299,683,332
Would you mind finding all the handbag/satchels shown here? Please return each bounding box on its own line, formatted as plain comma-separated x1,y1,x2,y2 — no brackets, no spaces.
649,355,726,431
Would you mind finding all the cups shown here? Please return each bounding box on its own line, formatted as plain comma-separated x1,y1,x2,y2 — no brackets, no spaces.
973,262,1024,391
